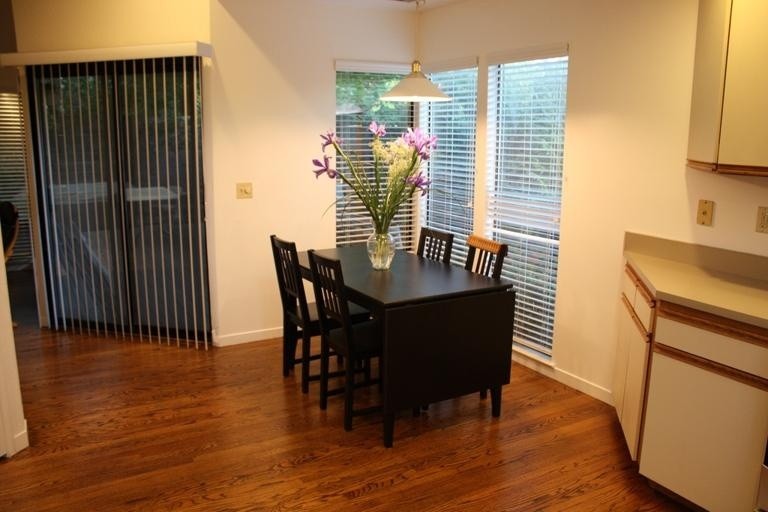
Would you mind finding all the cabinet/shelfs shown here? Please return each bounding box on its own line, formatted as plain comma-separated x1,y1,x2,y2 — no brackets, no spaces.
684,0,768,177
610,263,768,512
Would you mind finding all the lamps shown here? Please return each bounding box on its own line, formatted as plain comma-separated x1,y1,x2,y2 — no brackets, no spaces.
380,2,453,102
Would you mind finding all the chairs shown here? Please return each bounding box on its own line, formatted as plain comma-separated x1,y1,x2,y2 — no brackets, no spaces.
416,226,453,264
424,235,506,411
269,227,368,394
307,248,421,431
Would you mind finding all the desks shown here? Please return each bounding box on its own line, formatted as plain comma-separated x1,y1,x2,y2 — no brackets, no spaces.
281,245,517,446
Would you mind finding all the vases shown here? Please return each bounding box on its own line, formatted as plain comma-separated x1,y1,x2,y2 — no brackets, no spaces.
367,219,396,271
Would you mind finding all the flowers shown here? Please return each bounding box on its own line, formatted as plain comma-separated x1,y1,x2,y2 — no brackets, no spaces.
311,120,468,268
